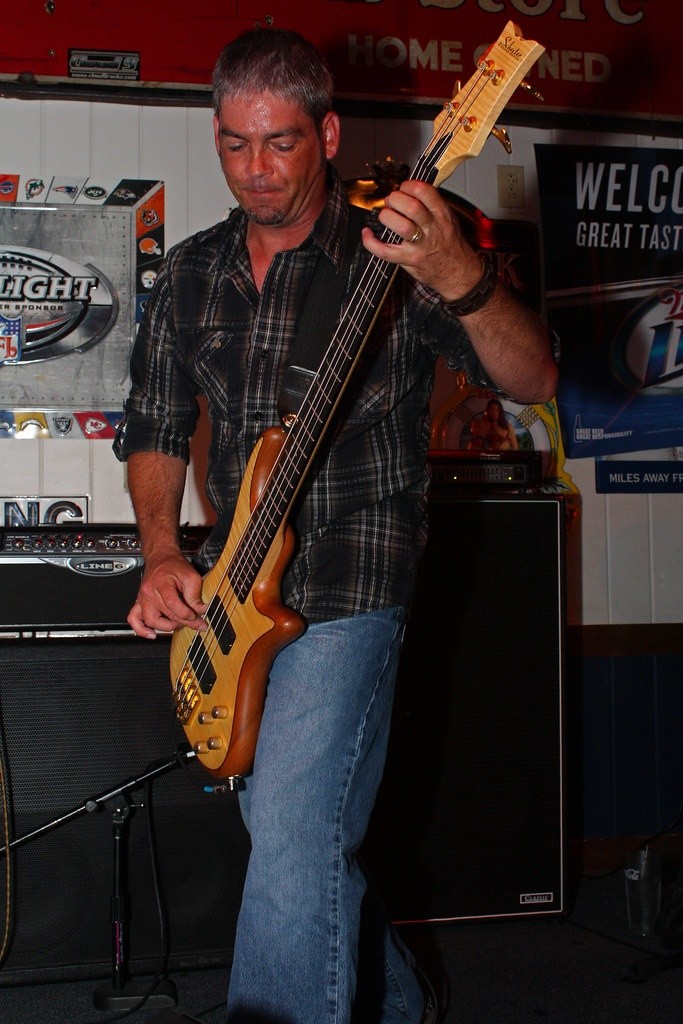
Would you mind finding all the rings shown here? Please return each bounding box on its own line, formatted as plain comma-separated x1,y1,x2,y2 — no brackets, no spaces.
408,227,421,244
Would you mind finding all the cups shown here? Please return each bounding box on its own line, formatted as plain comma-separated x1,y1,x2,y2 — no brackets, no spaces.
624,849,662,935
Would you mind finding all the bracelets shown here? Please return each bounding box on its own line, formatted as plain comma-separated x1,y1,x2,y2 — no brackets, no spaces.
442,252,499,317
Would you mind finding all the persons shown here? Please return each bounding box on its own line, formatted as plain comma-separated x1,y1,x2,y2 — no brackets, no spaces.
462,398,518,449
113,26,559,1024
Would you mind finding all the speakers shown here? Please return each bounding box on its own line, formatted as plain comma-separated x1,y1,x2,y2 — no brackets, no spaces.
360,492,569,934
0,628,257,985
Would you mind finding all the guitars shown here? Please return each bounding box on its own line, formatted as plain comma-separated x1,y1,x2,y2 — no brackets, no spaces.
168,20,547,792
